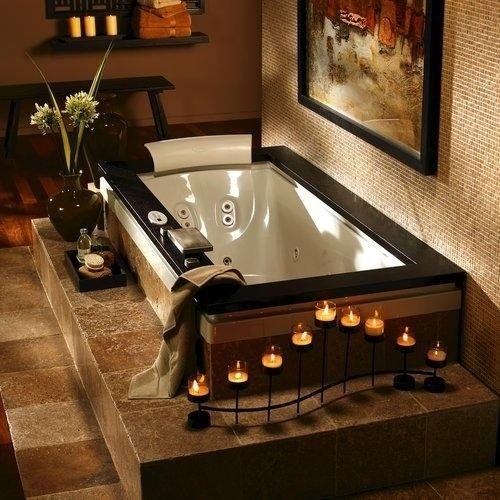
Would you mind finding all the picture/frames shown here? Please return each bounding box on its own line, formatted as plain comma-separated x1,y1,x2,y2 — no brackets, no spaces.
296,0,448,183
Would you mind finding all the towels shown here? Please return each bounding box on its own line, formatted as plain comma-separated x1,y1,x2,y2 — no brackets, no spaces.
127,262,247,405
136,0,194,39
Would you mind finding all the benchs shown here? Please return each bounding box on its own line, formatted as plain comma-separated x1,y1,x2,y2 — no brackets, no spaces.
0,75,178,159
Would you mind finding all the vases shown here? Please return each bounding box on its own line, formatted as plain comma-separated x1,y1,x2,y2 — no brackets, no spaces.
85,93,129,163
47,166,102,243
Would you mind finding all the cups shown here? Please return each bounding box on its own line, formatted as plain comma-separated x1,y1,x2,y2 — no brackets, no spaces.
288,322,313,346
104,11,118,36
313,300,385,337
396,326,416,346
187,374,210,396
227,360,248,382
83,13,96,38
426,341,447,360
83,255,104,273
262,345,284,369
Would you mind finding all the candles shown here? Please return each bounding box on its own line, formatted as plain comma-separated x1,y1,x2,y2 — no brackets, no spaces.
84,15,97,38
183,296,450,405
106,14,118,36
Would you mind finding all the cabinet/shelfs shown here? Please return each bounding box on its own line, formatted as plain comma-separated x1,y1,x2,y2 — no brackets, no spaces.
44,0,211,54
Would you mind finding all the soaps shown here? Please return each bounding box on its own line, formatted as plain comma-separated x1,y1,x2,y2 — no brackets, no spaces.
85,252,105,267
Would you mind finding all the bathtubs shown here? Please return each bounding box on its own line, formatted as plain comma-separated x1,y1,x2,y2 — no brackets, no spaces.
136,160,418,287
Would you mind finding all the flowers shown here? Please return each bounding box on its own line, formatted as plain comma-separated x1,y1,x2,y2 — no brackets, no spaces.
21,34,116,168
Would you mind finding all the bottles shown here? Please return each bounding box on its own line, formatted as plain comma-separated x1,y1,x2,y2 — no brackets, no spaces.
77,228,92,260
